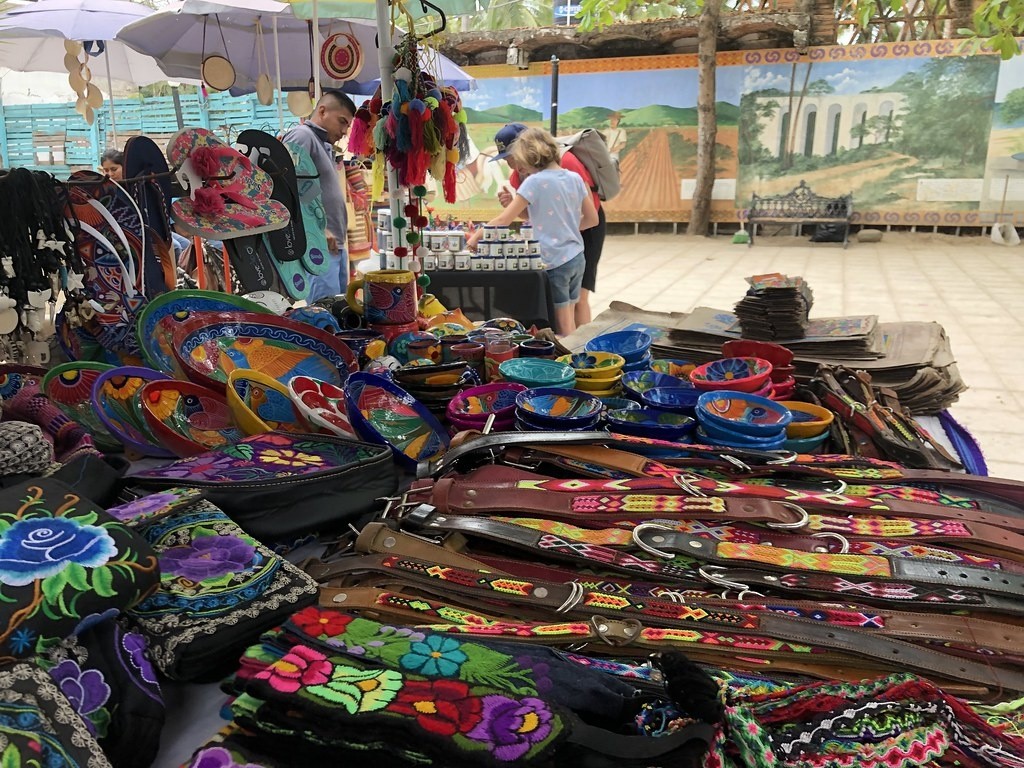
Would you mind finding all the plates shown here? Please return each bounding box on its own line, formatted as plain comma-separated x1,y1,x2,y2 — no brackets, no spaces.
0,289,833,471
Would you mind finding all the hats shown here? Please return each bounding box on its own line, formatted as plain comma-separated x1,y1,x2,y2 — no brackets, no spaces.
489,123,527,163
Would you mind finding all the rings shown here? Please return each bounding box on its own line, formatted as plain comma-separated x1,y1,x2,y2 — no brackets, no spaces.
471,249,476,252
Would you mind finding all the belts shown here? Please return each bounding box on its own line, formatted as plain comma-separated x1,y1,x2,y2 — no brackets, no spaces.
293,415,1023,702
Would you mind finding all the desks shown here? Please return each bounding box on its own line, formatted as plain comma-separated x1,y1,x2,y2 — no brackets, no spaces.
420,270,556,332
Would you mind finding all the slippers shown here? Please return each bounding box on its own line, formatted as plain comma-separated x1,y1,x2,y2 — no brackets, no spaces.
35,130,337,357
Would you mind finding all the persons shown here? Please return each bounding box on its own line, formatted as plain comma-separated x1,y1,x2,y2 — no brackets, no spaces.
464,125,599,338
278,89,359,307
100,149,126,182
488,121,606,330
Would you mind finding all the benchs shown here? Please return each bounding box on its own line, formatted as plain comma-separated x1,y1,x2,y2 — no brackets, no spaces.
747,178,855,249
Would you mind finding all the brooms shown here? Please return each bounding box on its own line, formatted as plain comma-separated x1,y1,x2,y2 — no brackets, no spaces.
731,156,750,245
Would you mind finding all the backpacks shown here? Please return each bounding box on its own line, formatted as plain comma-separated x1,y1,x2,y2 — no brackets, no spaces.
561,128,620,201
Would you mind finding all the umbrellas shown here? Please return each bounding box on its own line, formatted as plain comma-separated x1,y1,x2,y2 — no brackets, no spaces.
113,0,408,140
0,0,207,151
228,41,480,98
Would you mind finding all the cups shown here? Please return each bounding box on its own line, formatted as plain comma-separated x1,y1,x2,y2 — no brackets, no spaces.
344,267,419,324
